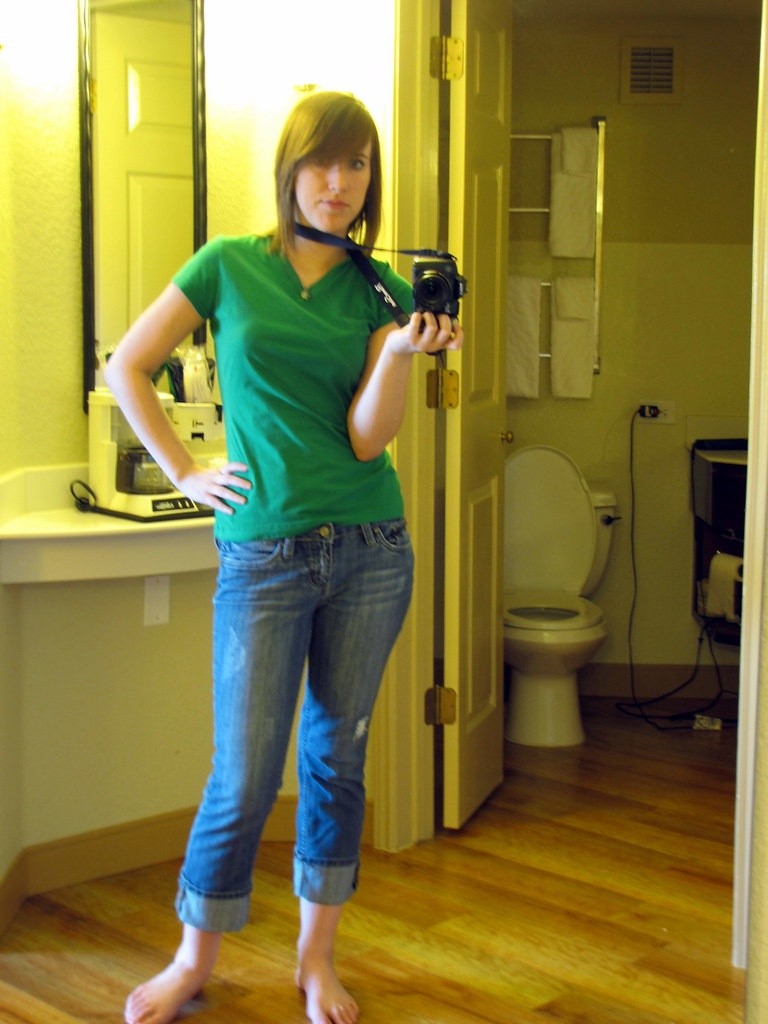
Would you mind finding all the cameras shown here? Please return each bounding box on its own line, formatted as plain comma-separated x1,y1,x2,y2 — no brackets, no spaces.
412,255,466,335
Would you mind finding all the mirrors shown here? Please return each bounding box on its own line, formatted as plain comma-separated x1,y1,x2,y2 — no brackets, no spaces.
76,0,207,416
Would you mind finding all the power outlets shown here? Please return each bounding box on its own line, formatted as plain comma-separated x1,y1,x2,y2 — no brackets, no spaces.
638,399,677,425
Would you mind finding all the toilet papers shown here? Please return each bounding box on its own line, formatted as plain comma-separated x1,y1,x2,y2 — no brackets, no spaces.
706,554,744,620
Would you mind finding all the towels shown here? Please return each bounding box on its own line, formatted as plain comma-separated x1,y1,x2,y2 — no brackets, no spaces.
503,275,540,399
549,126,598,258
549,277,596,400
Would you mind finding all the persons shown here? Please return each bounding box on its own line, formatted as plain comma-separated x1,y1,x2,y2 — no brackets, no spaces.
103,93,465,1024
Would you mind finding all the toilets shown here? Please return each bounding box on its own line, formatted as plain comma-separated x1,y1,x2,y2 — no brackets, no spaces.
502,443,618,749
